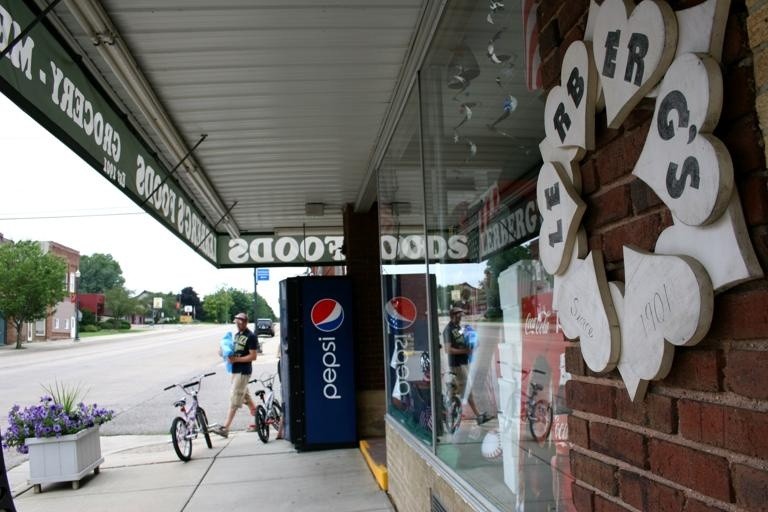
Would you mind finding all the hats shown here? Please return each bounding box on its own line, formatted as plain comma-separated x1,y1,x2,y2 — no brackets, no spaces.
449,307,464,316
234,311,248,320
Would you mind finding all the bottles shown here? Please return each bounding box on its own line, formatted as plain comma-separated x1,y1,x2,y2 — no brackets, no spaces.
522,346,569,512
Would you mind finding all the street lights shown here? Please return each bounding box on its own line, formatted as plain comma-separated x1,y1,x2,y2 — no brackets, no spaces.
74,268,81,339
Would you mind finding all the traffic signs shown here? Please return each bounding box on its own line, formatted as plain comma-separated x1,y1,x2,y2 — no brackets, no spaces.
257,269,269,281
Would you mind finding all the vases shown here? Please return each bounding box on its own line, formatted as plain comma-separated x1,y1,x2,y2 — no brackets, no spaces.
24,422,104,494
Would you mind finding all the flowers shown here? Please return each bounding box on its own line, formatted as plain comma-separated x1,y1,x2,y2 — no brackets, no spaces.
0,377,113,453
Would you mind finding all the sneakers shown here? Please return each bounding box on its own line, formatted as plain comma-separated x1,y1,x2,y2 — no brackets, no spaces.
211,422,229,438
477,413,495,425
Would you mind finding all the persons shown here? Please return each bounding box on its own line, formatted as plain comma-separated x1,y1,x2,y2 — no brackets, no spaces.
274,340,285,440
212,312,258,437
442,307,494,425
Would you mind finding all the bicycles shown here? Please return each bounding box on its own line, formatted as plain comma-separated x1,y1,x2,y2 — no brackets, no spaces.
442,372,460,433
497,361,552,442
249,373,281,443
164,372,212,461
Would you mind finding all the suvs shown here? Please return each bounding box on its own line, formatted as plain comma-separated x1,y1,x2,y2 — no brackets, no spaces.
254,318,274,336
